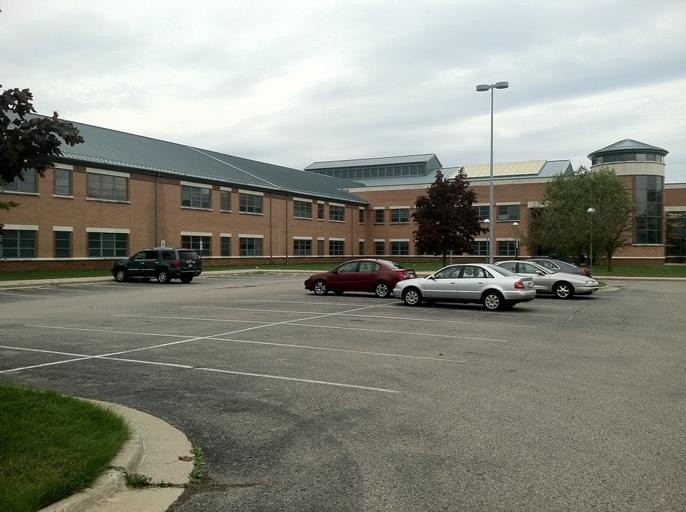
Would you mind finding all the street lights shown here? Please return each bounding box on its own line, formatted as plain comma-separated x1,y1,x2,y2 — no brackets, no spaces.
513,222,520,260
587,207,595,268
483,219,491,264
476,81,509,265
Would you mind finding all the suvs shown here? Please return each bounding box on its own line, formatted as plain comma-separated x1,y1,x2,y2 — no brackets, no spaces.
111,247,203,284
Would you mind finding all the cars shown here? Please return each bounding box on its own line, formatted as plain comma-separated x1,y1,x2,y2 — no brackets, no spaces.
391,256,603,311
305,258,418,299
